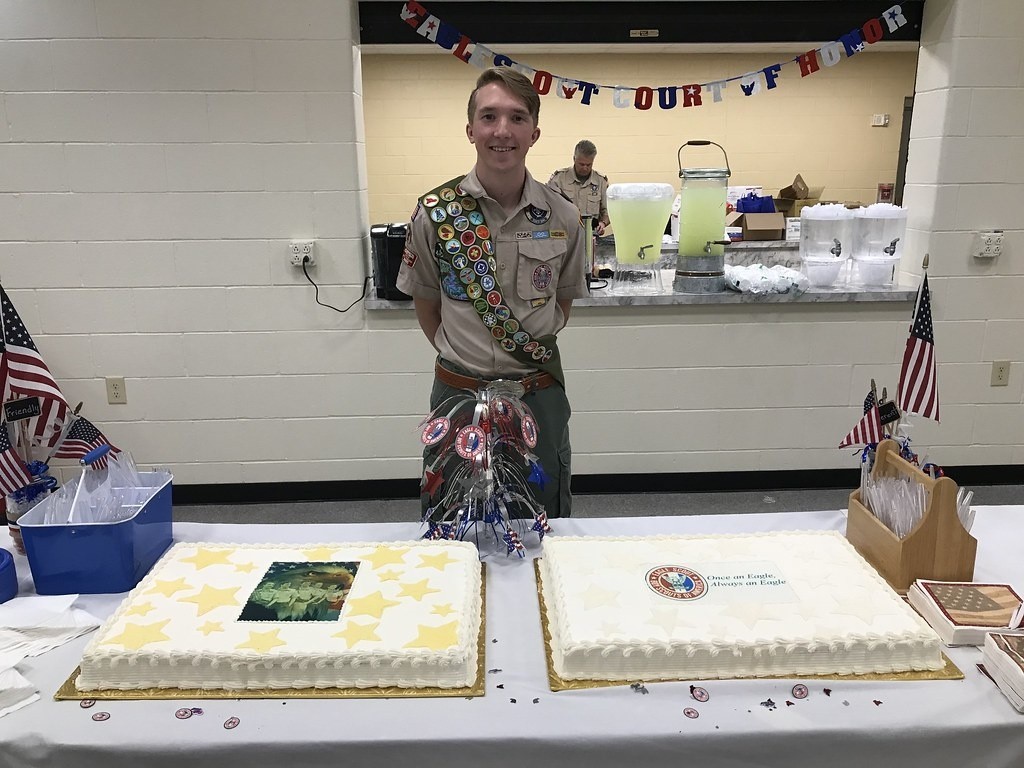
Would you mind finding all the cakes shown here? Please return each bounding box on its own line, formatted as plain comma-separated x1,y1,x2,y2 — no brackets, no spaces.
74,539,482,693
538,531,947,680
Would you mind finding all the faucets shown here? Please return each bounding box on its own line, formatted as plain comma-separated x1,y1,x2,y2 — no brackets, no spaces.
703,240,732,255
832,238,842,258
637,244,654,260
885,237,900,256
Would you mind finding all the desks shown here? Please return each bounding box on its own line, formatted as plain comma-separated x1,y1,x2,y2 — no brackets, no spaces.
0,504,1024,767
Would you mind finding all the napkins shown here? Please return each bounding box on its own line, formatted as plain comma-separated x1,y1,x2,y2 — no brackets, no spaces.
0,593,103,716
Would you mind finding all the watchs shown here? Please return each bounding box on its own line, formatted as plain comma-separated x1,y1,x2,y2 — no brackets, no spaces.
600,218,607,227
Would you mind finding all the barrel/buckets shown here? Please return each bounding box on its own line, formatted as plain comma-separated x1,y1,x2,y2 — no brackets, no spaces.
677,140,732,276
798,217,854,289
606,182,676,266
852,216,907,289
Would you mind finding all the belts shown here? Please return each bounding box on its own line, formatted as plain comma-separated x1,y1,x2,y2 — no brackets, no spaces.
435,361,554,399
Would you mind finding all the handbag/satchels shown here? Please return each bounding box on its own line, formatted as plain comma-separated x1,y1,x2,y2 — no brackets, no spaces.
737,192,776,213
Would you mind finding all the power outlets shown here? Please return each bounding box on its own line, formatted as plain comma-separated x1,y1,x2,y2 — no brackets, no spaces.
973,232,1004,258
991,361,1010,387
105,376,127,405
290,240,315,268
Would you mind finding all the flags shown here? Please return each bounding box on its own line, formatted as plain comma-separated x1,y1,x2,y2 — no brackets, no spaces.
837,272,942,447
0,282,126,499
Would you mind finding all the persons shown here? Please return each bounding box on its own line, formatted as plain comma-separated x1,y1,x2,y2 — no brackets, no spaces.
547,140,611,235
397,66,588,522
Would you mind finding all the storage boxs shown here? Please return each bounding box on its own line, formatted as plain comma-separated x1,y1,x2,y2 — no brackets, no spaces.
16,472,173,595
774,175,825,217
725,210,786,240
